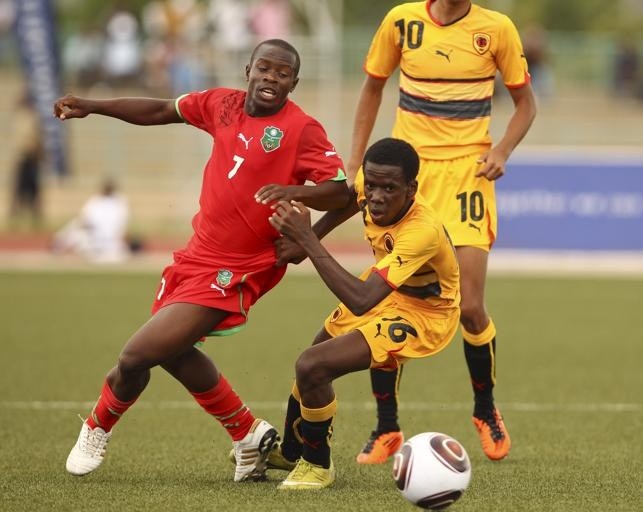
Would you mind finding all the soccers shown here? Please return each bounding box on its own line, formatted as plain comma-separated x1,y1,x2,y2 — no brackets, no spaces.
394,432,472,510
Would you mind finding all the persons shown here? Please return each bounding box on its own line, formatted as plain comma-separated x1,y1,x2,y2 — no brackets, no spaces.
229,138,462,490
348,0,536,464
53,39,350,482
11,0,293,265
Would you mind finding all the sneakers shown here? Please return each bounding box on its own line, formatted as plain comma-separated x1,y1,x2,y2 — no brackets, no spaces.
472,407,511,460
356,429,403,464
277,456,336,490
229,442,299,471
233,419,278,483
66,417,112,476
